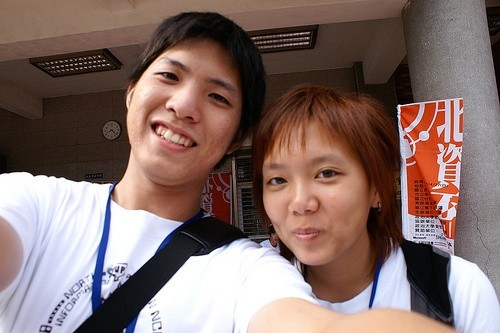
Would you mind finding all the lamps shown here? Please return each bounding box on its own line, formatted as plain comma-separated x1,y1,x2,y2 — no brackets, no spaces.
245,24,317,53
29,48,124,80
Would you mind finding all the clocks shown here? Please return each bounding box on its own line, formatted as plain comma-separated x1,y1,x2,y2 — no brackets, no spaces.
102,121,121,142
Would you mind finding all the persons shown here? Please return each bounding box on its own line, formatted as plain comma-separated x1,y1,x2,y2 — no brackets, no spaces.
0,11,457,333
251,83,500,333
259,224,281,256
